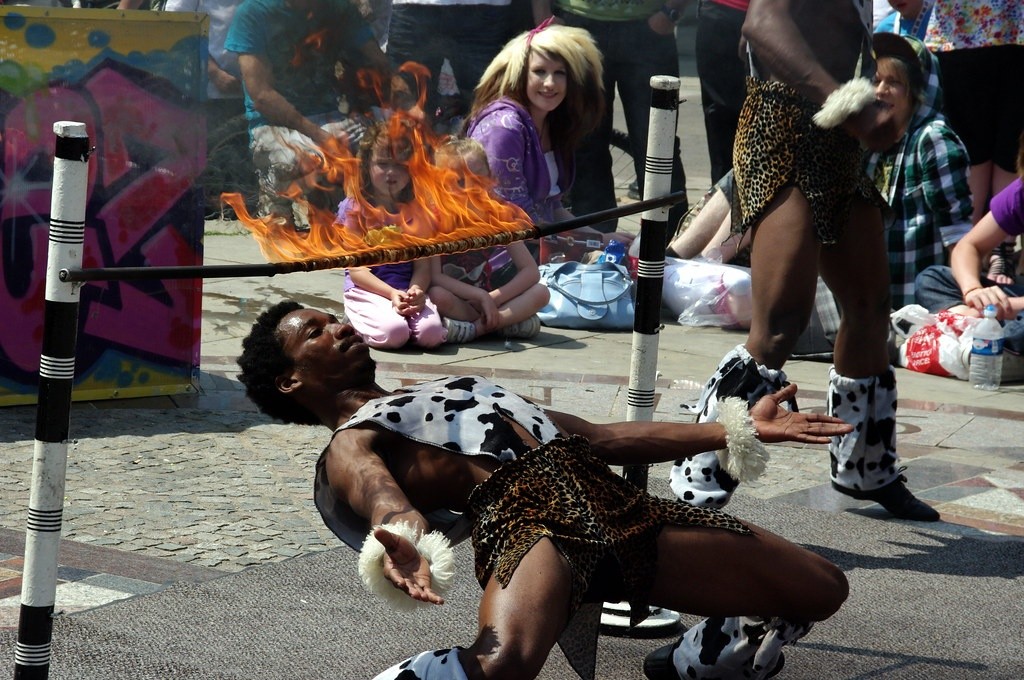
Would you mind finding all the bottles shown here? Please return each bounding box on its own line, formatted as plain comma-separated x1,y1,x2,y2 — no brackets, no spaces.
968,305,1004,390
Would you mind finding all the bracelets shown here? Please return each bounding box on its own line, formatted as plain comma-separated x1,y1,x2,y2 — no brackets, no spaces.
812,76,877,129
714,395,770,483
962,287,980,298
357,519,456,612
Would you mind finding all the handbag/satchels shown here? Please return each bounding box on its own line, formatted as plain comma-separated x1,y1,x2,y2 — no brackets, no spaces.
891,303,982,378
537,260,635,329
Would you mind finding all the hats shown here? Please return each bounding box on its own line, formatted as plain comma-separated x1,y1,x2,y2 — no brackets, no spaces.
872,32,932,87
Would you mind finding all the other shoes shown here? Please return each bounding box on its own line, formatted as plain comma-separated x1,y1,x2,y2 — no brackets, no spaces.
962,341,1024,382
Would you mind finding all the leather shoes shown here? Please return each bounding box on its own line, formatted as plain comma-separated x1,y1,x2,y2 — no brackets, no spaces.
832,466,941,521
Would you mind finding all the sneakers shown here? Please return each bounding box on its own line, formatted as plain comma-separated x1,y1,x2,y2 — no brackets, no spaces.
443,317,476,343
643,636,683,680
499,313,540,339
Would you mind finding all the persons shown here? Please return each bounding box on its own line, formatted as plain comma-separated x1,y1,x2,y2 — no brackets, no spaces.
236,301,854,680
115,0,1024,380
668,0,941,522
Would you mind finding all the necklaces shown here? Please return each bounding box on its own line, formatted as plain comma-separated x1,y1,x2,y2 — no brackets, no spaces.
538,119,545,142
